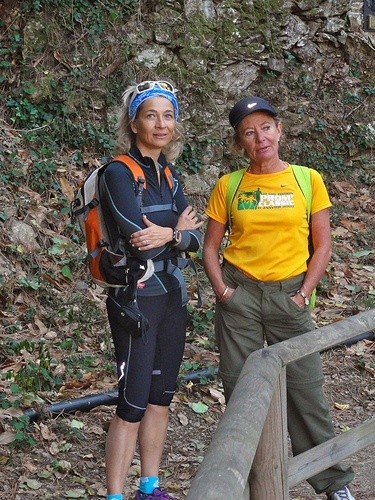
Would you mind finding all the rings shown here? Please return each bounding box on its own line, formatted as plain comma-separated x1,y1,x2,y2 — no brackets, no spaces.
145,240,148,245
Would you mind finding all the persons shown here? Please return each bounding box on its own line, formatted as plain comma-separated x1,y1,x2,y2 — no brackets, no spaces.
104,79,205,500
201,95,356,500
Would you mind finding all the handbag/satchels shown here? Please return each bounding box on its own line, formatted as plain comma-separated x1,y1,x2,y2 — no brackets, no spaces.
116,308,148,337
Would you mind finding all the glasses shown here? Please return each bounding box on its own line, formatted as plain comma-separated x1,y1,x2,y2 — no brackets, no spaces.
129,80,175,106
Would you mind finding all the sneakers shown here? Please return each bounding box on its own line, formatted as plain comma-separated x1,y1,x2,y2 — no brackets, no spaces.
330,486,356,500
135,487,178,500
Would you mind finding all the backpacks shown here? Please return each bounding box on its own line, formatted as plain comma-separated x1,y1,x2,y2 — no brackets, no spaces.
70,155,186,288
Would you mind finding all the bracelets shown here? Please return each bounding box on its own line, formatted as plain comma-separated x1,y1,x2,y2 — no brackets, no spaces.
217,285,229,304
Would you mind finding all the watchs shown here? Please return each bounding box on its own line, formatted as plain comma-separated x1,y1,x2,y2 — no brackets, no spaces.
164,226,180,248
296,289,311,307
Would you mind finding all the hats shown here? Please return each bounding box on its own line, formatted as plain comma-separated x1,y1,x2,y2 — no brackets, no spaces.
229,96,278,128
